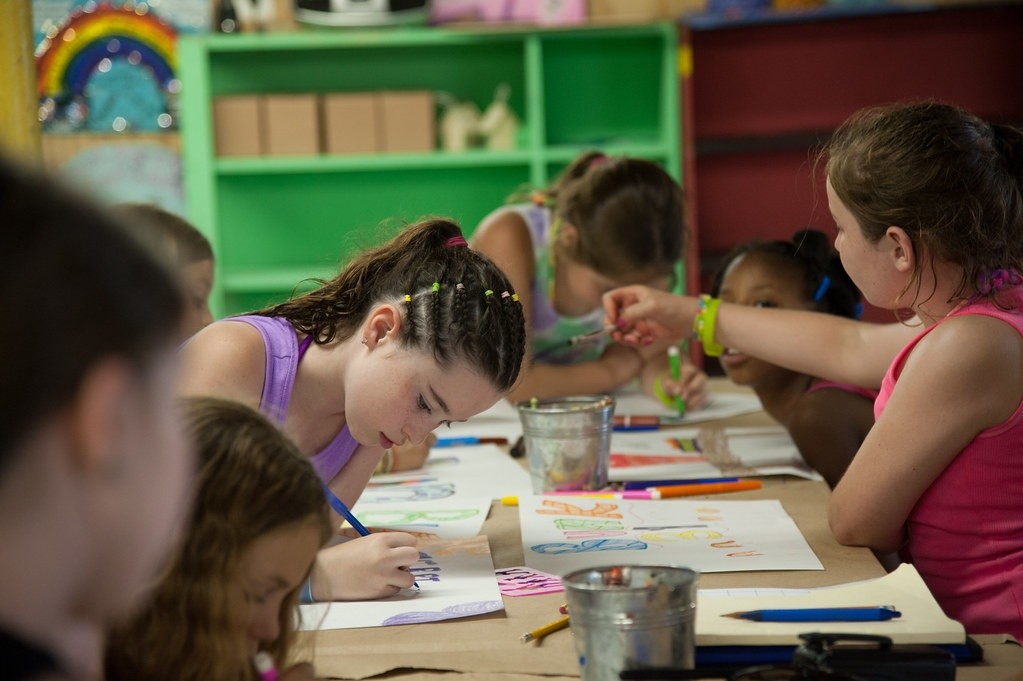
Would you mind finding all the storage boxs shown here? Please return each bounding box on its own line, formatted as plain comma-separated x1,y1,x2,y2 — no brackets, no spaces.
324,92,377,154
262,94,319,157
214,97,260,155
380,91,434,151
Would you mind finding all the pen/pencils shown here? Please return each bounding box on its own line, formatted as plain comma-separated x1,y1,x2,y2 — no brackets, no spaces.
519,603,571,645
612,416,659,432
567,324,618,347
668,346,684,418
323,487,419,588
718,606,902,623
434,436,525,458
545,477,762,500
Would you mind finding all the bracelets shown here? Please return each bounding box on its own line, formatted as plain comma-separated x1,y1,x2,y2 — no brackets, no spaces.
693,296,724,356
655,370,675,407
300,578,313,604
385,447,397,473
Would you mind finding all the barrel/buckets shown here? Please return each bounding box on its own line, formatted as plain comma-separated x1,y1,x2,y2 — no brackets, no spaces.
517,393,617,492
561,564,701,681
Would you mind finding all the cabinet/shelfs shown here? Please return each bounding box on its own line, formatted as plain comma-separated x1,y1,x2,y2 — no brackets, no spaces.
679,0,1023,370
175,21,680,328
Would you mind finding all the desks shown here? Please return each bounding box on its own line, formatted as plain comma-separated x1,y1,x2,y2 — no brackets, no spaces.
287,378,888,681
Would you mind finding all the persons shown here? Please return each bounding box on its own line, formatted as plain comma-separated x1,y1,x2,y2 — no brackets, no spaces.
114,201,436,476
0,153,194,681
176,220,526,603
105,397,333,681
602,103,1023,649
709,228,878,491
468,152,707,410
